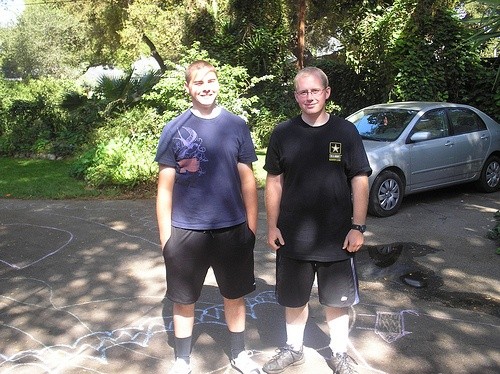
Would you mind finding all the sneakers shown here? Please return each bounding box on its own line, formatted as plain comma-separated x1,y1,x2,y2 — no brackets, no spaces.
330,350,358,374
263,343,304,374
232,350,262,374
168,357,192,374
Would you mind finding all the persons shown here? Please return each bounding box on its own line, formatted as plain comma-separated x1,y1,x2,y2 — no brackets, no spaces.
155,60,262,374
261,64,375,374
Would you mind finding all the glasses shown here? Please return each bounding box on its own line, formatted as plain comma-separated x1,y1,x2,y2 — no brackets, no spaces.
295,87,326,97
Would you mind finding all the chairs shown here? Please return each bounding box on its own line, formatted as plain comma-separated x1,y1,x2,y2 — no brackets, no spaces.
415,119,444,138
453,116,478,135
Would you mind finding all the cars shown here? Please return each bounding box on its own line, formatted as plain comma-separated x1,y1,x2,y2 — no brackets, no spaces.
345,101,500,217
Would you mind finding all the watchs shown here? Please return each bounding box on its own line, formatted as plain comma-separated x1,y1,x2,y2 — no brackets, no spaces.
351,223,366,233
